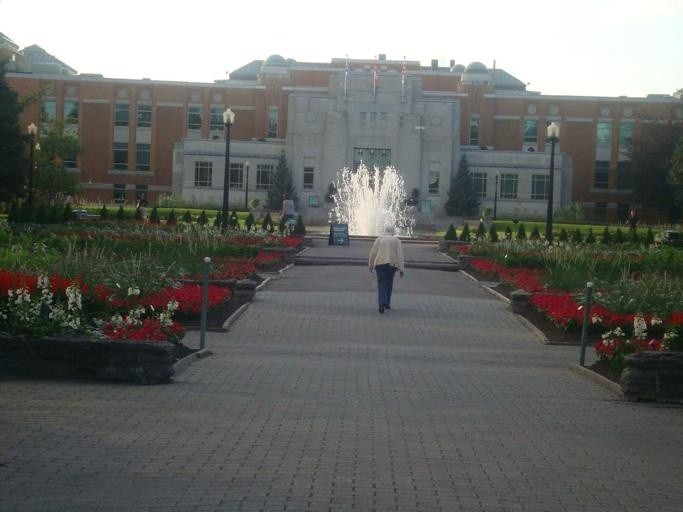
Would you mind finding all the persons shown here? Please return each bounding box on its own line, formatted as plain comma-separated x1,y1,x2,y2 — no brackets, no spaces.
279,194,296,214
369,224,405,313
625,209,640,231
136,192,147,221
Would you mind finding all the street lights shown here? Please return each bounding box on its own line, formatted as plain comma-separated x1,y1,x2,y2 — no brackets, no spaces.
217,105,236,229
25,121,38,202
243,159,249,210
543,120,561,242
493,171,500,219
